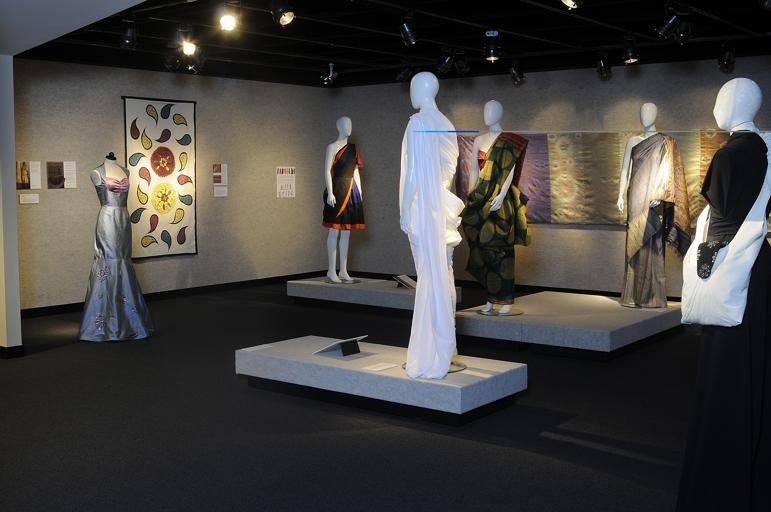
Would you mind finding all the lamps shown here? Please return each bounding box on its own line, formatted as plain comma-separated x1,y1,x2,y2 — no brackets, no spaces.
485,42,500,63
401,21,420,46
508,64,526,86
595,56,613,81
563,0,580,9
719,46,736,75
177,40,204,75
216,13,239,34
621,37,638,63
437,54,453,75
655,11,678,40
321,61,339,87
269,3,296,27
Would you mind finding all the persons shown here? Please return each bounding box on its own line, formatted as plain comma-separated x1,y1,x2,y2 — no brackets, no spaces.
398,69,469,380
461,99,533,315
676,77,771,509
78,150,157,343
21,161,29,189
616,102,695,309
320,115,367,284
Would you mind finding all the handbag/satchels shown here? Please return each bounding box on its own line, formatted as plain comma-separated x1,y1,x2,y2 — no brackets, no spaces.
681,232,767,326
